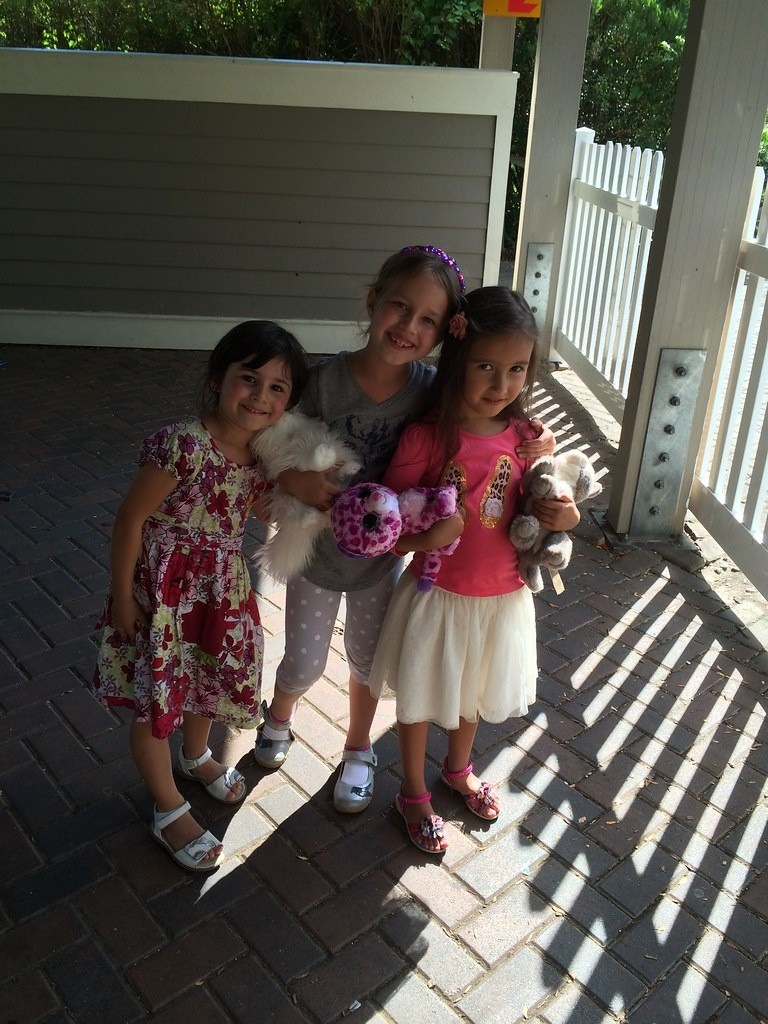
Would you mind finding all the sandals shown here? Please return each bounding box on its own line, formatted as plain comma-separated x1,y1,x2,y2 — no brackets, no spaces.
396,781,450,854
148,800,225,872
176,743,247,804
440,755,502,820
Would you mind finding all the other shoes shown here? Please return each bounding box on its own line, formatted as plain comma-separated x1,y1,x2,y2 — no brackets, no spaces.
334,745,378,814
254,699,300,769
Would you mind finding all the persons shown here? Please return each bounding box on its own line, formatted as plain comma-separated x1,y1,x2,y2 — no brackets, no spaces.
88,320,309,873
250,244,467,813
366,286,581,854
